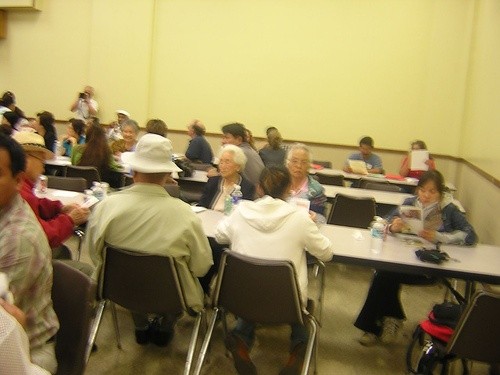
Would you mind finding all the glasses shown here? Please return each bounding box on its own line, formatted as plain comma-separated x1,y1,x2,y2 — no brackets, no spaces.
26,152,46,168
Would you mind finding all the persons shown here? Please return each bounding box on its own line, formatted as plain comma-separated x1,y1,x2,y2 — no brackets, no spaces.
86,133,214,347
399,140,438,193
215,165,335,375
343,136,384,188
144,118,327,307
0,85,139,375
353,170,478,347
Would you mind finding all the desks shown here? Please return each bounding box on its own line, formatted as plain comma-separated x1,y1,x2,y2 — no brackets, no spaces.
43,153,500,375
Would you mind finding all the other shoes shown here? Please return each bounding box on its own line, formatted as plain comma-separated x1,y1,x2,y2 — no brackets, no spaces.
382,319,404,343
228,335,257,375
136,328,151,345
152,329,169,346
278,343,307,375
360,334,377,345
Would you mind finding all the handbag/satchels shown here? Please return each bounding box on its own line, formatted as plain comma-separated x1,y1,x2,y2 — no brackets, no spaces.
433,303,461,329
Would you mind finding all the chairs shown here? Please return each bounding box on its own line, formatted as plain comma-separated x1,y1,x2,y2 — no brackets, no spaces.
51,256,94,375
42,163,67,177
192,249,318,375
325,194,377,230
85,243,203,375
363,182,403,193
412,290,500,375
65,164,102,190
52,222,85,261
315,173,345,187
45,176,87,194
351,177,390,189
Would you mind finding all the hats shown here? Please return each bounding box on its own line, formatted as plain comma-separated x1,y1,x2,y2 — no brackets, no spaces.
116,110,130,120
11,132,55,160
120,134,183,174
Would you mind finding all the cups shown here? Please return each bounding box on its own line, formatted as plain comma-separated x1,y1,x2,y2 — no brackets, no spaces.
39,175,48,193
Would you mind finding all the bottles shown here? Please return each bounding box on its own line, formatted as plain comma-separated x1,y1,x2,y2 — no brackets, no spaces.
230,185,243,206
92,182,104,200
369,217,384,255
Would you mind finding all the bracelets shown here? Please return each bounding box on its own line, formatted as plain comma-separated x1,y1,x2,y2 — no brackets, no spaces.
387,224,393,235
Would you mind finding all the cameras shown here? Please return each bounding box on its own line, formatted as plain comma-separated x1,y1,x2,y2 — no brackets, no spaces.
79,93,84,98
